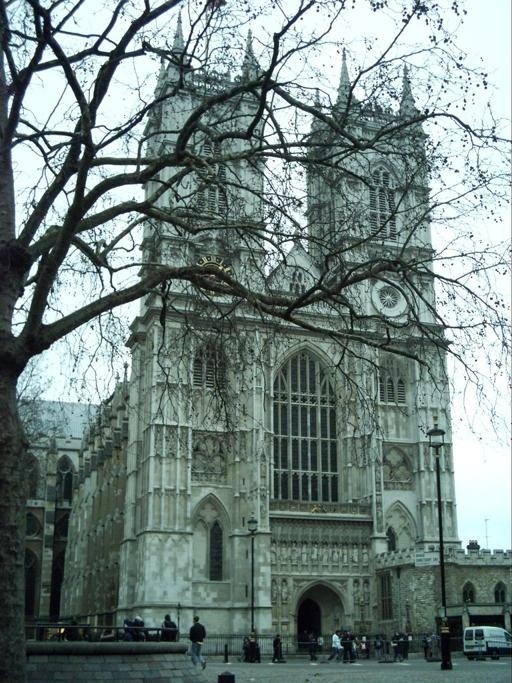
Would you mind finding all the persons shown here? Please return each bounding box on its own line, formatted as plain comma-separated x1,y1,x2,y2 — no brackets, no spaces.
242,630,281,663
299,629,408,663
61,615,207,670
422,631,439,658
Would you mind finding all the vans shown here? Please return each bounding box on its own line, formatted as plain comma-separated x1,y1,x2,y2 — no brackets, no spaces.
463,625,512,660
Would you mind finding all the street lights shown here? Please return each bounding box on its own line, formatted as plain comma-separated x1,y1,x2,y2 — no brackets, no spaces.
247,511,258,643
425,423,452,670
